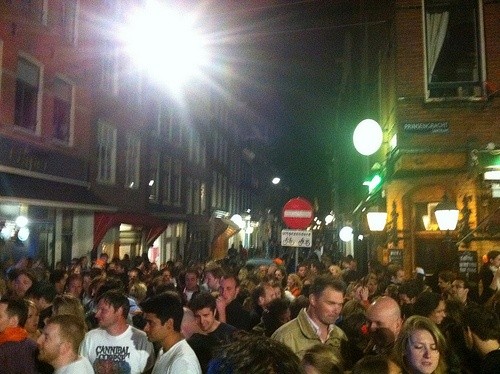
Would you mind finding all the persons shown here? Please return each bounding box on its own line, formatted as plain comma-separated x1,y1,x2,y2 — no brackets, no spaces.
0,241,500,374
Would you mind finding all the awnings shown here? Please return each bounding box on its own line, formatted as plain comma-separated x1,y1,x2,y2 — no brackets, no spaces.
0,166,118,213
149,204,187,219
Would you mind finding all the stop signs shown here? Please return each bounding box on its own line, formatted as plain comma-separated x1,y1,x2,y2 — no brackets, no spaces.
281,197,314,230
